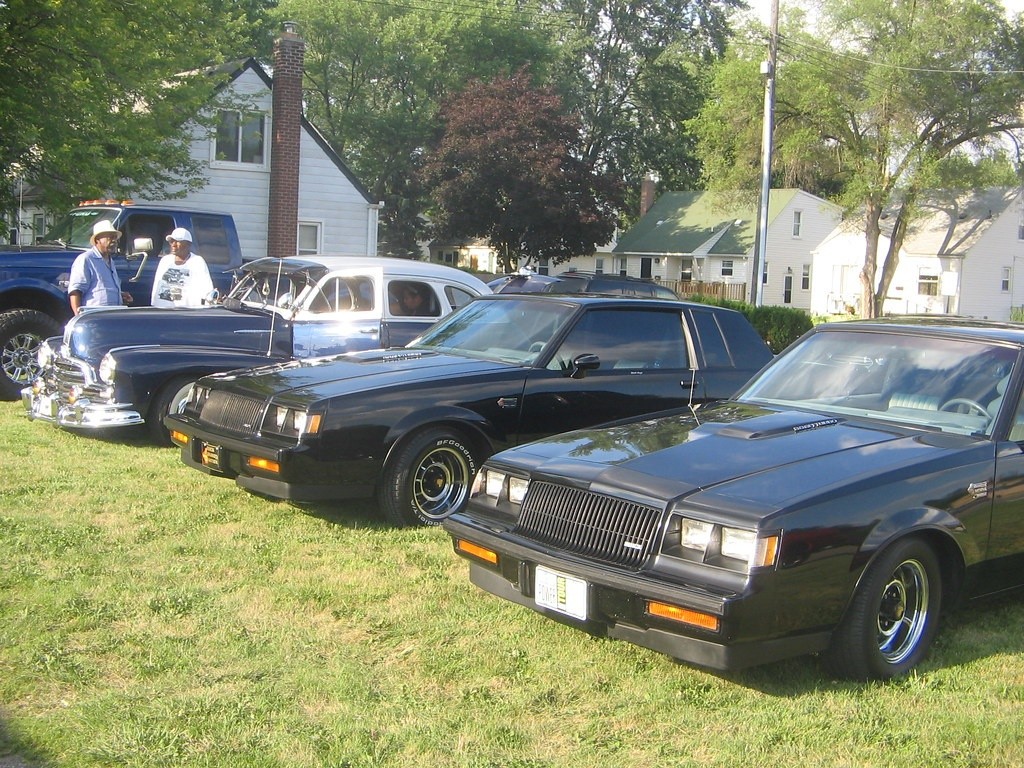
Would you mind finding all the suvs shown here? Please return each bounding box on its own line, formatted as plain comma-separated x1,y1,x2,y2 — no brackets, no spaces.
490,273,674,300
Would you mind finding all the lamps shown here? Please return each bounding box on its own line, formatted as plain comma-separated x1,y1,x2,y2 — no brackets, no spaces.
655,276,661,280
655,258,660,263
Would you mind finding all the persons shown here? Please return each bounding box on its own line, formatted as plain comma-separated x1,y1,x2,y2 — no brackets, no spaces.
390,285,433,316
68,220,133,315
151,228,214,307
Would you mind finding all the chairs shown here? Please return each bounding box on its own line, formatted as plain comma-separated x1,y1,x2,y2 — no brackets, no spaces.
986,373,1024,442
544,315,574,371
611,359,647,369
887,392,940,413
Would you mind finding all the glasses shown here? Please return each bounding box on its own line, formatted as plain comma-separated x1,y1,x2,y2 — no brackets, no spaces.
97,234,118,241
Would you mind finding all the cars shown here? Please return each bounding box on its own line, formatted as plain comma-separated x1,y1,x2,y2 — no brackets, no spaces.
40,252,486,427
169,292,774,529
444,323,1021,680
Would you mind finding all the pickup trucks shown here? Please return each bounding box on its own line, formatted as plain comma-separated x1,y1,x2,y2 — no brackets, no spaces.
0,201,253,398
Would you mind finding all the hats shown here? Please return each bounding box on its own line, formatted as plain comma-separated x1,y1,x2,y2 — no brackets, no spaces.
89,220,122,246
166,228,192,243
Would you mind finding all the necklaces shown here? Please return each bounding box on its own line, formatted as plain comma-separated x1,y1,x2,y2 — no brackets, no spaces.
182,254,189,264
